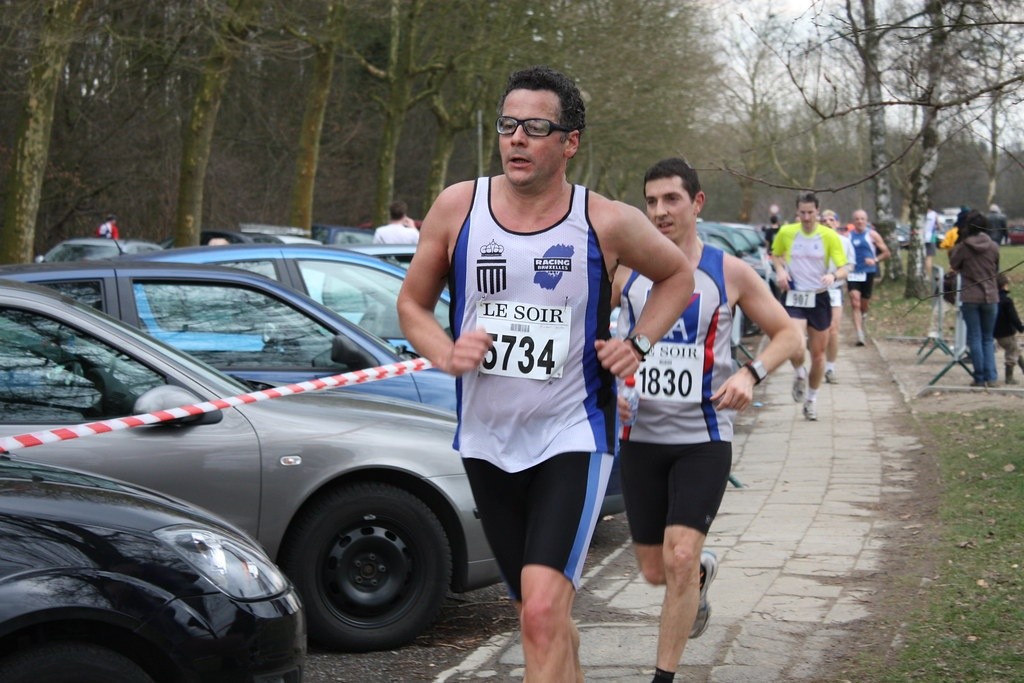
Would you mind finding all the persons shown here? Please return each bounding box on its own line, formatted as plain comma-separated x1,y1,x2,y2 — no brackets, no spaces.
396,67,694,683
771,192,848,421
815,209,891,385
95,214,118,240
940,204,1024,387
925,201,941,283
606,159,801,683
373,201,421,270
765,204,801,258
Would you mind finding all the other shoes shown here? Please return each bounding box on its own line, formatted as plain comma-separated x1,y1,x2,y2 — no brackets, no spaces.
803,400,817,420
1006,378,1019,384
991,381,1002,388
971,382,984,387
826,370,839,384
793,368,807,403
855,332,865,346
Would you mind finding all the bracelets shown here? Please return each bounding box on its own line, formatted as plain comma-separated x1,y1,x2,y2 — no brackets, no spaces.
832,272,837,281
874,258,879,263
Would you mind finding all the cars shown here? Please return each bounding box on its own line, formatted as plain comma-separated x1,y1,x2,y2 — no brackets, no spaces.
895,222,912,249
1,454,308,683
695,220,778,338
1004,219,1024,246
933,206,962,243
0,277,505,654
22,225,451,360
0,257,460,417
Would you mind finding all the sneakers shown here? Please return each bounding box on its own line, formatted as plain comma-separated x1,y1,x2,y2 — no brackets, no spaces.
689,552,719,639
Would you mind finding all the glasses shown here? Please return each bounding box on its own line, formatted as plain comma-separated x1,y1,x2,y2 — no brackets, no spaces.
495,116,572,136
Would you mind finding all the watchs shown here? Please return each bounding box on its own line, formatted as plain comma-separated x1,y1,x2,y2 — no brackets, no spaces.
626,333,651,355
743,359,767,384
